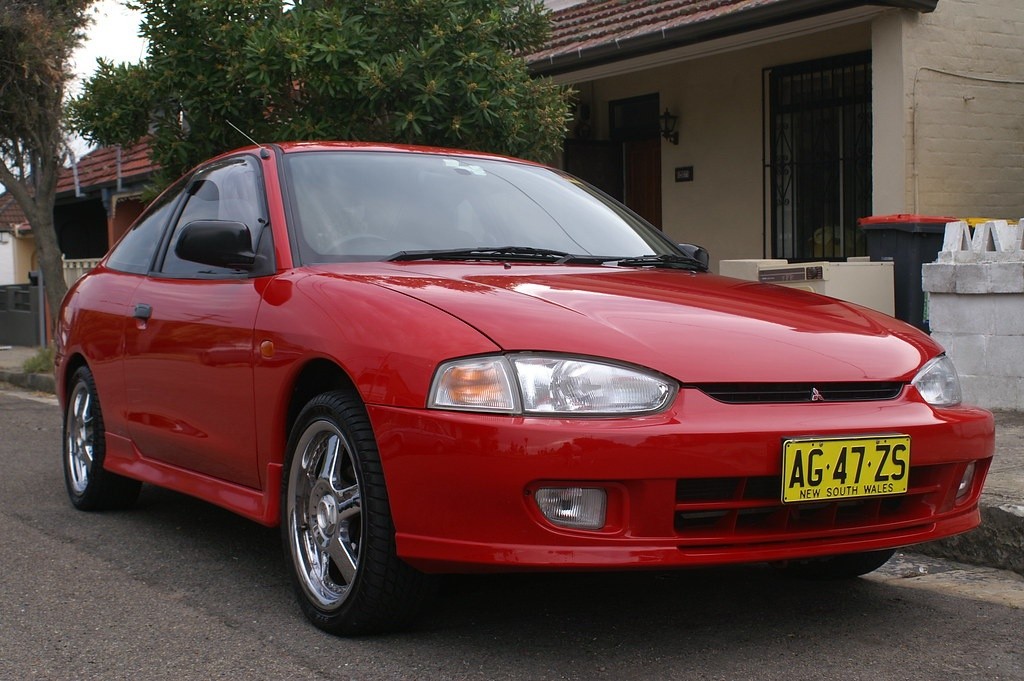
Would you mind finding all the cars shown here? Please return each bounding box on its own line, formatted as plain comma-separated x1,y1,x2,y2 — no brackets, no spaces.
46,137,998,638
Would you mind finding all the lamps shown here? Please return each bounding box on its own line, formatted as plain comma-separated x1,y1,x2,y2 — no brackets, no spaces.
658,107,681,146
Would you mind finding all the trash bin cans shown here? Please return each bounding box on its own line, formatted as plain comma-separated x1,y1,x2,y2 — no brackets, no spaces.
860,215,961,337
959,218,1021,242
